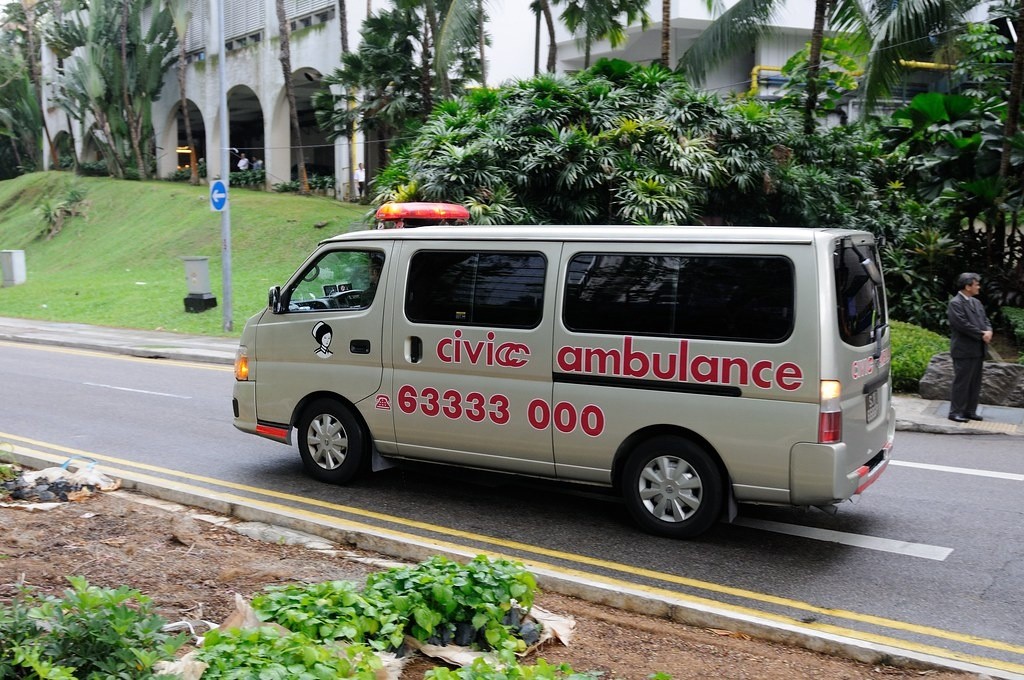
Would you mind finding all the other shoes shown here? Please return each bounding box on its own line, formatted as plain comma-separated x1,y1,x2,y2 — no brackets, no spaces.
960,414,983,421
949,413,970,422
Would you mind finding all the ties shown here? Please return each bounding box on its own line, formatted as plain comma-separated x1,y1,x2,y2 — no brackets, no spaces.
967,299,975,307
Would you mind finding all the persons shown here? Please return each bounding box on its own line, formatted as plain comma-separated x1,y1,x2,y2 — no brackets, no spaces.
237,152,263,170
360,256,385,307
948,272,993,422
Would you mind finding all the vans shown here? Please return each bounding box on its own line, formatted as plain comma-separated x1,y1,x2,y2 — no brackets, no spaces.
228,201,897,540
